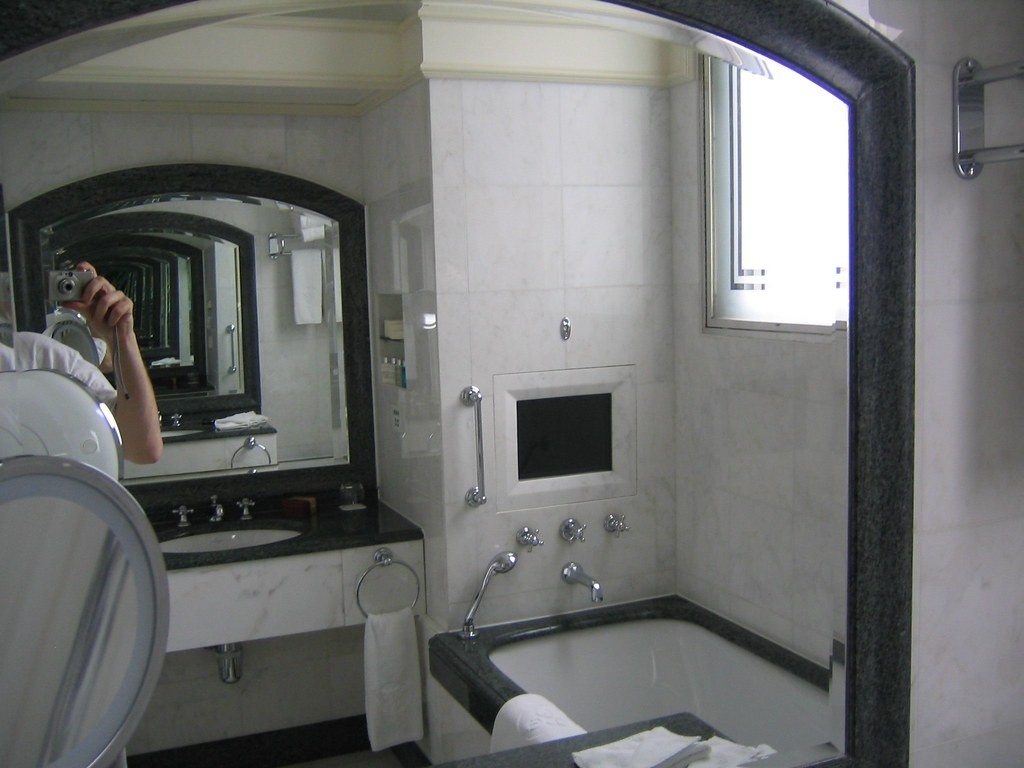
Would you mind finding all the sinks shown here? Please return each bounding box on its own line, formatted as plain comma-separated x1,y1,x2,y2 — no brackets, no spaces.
160,428,206,438
156,525,302,554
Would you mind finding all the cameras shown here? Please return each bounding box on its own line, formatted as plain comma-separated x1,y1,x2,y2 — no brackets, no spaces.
44,270,94,301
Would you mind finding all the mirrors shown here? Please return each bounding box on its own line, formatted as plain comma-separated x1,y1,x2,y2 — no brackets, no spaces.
4,162,379,516
34,209,262,421
0,0,920,768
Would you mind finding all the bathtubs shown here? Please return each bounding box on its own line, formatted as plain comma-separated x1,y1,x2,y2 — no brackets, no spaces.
420,592,832,766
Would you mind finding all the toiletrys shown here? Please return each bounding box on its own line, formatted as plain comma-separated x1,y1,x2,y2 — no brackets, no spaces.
382,357,407,389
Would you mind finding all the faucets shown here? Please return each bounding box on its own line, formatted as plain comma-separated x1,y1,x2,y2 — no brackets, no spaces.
208,494,224,522
568,564,604,604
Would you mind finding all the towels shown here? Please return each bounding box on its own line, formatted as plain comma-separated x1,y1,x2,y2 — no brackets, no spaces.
214,409,270,431
290,250,323,326
151,356,181,366
489,692,587,758
363,605,424,753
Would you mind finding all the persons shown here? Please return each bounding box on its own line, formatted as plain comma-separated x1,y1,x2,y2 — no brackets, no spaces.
0,262,163,463
